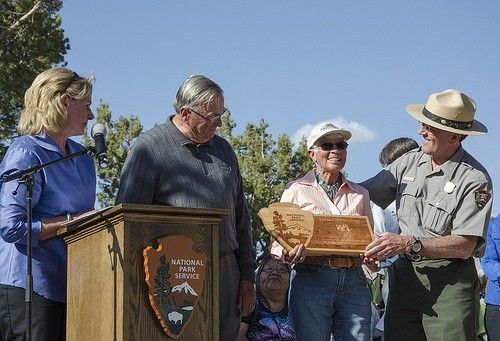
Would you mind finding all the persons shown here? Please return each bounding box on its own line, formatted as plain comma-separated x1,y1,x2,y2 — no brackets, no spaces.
356,89,493,341
114,75,258,341
0,68,96,341
370,137,500,341
237,254,296,341
270,121,374,341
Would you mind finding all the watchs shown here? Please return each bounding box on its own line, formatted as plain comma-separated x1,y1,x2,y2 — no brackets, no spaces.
410,236,422,254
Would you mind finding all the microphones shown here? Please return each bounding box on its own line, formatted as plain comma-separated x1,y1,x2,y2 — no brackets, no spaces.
92,123,108,168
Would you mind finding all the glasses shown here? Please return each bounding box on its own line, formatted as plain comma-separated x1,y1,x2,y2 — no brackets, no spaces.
61,72,82,95
187,108,226,123
262,267,288,273
418,121,434,134
315,142,349,150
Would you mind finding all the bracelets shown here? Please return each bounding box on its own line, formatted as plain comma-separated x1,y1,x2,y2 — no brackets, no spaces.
67,212,72,221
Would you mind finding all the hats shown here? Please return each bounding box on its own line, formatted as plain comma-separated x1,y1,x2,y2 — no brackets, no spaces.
405,88,488,134
307,123,352,150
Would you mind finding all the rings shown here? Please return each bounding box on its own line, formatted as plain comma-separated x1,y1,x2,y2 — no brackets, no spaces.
298,257,304,263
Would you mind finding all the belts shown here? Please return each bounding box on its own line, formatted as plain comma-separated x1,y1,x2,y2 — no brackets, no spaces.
302,256,362,269
